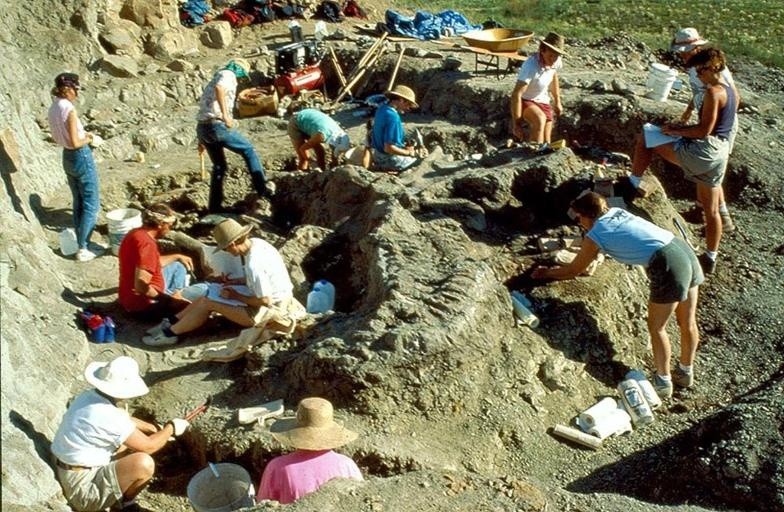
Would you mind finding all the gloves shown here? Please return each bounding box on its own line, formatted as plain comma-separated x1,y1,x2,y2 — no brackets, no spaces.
165,417,191,435
409,147,428,159
88,134,103,147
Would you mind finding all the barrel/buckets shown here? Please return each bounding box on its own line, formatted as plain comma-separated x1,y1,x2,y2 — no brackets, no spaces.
644,64,679,101
187,463,259,512
304,288,328,312
106,208,143,257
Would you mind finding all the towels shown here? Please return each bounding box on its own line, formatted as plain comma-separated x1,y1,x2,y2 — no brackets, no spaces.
625,369,662,411
618,379,654,429
588,409,633,440
576,397,618,431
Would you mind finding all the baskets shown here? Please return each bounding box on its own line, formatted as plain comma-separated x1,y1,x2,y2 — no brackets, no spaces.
461,28,535,52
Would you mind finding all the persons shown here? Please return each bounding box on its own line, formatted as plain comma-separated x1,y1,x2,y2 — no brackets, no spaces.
196,56,271,214
49,355,192,512
140,217,294,346
371,83,428,169
622,47,740,274
286,109,372,172
255,397,364,504
510,32,566,145
530,190,704,398
47,72,106,262
671,26,741,234
116,204,209,325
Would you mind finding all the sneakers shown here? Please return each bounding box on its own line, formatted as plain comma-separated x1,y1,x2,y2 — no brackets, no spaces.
77,249,97,261
670,367,693,387
682,205,734,274
142,332,178,346
650,375,673,399
613,177,636,202
146,317,172,335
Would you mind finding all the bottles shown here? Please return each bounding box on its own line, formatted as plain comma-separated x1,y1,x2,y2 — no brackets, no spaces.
306,288,328,313
60,226,79,256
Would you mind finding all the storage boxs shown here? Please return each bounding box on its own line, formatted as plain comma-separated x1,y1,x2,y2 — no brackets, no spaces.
236,84,279,116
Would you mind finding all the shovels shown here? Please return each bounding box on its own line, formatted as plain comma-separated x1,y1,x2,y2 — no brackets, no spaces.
321,54,378,113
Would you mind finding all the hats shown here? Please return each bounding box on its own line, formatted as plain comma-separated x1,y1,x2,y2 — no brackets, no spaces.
330,132,349,158
538,31,569,56
270,396,359,451
55,73,86,90
212,217,254,255
670,27,708,53
85,357,150,399
231,57,251,84
385,84,420,112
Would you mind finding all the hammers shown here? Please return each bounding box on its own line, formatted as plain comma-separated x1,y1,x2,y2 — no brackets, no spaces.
673,218,701,253
184,394,212,421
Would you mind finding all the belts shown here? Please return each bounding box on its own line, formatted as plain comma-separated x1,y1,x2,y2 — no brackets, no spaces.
198,120,223,124
712,132,729,141
56,460,92,470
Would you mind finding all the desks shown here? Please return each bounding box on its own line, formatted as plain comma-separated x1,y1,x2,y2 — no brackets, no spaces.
461,46,528,80
